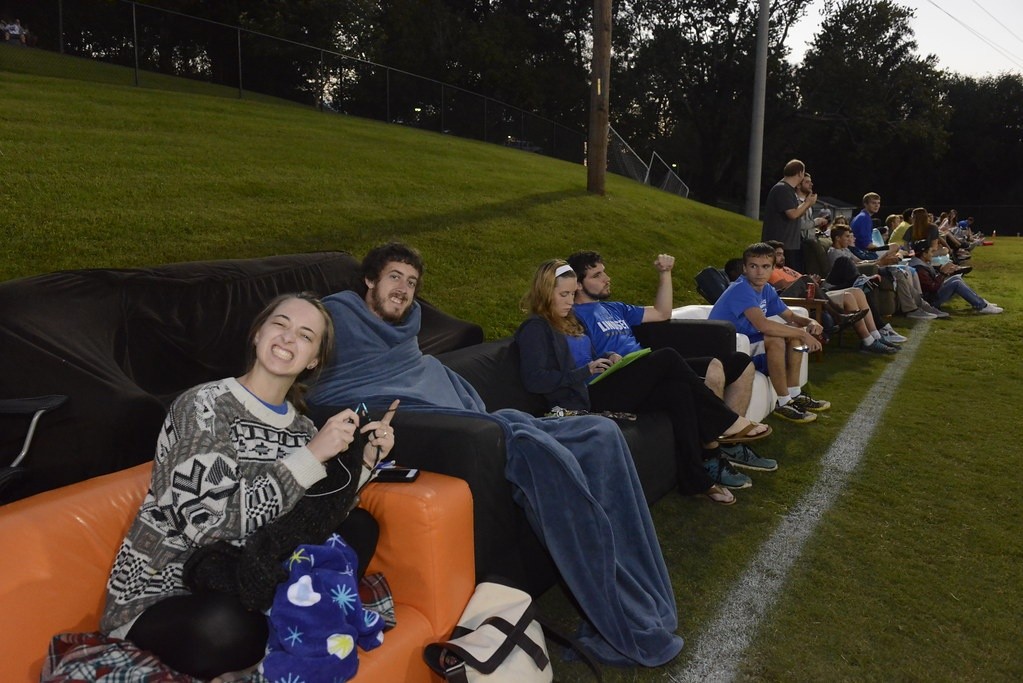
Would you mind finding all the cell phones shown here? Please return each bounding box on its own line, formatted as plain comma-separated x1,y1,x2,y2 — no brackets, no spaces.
370,469,419,483
793,344,809,352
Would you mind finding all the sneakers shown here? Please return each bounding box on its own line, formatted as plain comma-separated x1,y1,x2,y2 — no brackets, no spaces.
920,302,950,318
975,299,1005,314
794,391,832,412
881,326,908,343
860,339,895,355
701,453,752,489
774,398,817,424
906,308,938,320
720,443,778,472
878,334,902,351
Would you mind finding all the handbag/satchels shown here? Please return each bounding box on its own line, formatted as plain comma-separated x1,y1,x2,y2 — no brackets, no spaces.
423,581,553,683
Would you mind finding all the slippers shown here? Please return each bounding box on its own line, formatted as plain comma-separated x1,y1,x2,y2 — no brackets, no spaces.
694,483,736,505
714,417,771,443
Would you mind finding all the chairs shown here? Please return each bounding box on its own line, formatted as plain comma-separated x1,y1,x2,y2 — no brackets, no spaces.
779,279,843,362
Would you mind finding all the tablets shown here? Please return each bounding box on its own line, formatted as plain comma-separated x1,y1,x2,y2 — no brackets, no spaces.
589,348,651,385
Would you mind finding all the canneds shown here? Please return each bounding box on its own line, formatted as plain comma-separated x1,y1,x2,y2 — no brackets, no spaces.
807,283,815,300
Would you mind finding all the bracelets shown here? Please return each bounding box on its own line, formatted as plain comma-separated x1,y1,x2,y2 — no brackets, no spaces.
362,461,376,475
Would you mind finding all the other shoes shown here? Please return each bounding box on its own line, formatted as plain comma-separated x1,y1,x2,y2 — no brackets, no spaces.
838,305,869,329
958,253,971,260
949,267,973,277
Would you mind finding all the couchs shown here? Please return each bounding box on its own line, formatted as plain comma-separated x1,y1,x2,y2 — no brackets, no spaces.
304,318,736,597
0,459,476,683
802,237,899,318
671,305,809,422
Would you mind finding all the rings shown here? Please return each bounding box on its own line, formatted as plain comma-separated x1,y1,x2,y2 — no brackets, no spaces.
383,432,387,437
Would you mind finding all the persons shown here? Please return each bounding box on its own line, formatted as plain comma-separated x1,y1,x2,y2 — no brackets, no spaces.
820,256,906,342
827,225,949,318
825,215,847,236
847,229,923,297
99,291,400,683
515,258,772,505
819,204,831,217
878,207,986,274
795,173,828,239
762,160,817,271
765,240,902,355
724,257,869,326
708,243,830,423
567,251,778,489
909,240,1003,313
300,242,616,588
850,193,897,252
